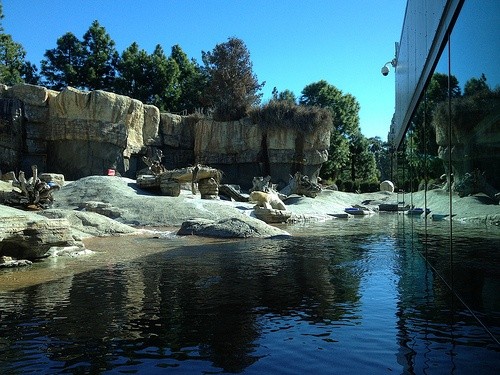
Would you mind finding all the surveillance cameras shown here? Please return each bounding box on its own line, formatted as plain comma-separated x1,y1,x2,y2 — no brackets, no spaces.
381,66,390,76
